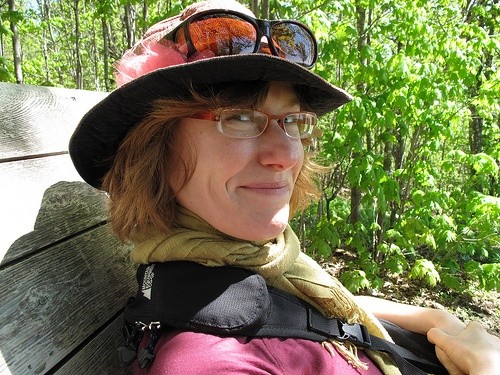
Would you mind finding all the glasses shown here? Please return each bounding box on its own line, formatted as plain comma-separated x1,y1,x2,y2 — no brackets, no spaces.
178,107,318,139
157,8,318,71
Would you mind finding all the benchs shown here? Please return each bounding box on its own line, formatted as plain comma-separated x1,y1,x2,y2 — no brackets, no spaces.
0,82,140,373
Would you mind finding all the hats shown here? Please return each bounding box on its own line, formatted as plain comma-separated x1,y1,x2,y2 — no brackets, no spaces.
69,0,355,191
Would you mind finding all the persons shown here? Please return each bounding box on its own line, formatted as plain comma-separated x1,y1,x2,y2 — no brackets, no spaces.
68,1,500,375
